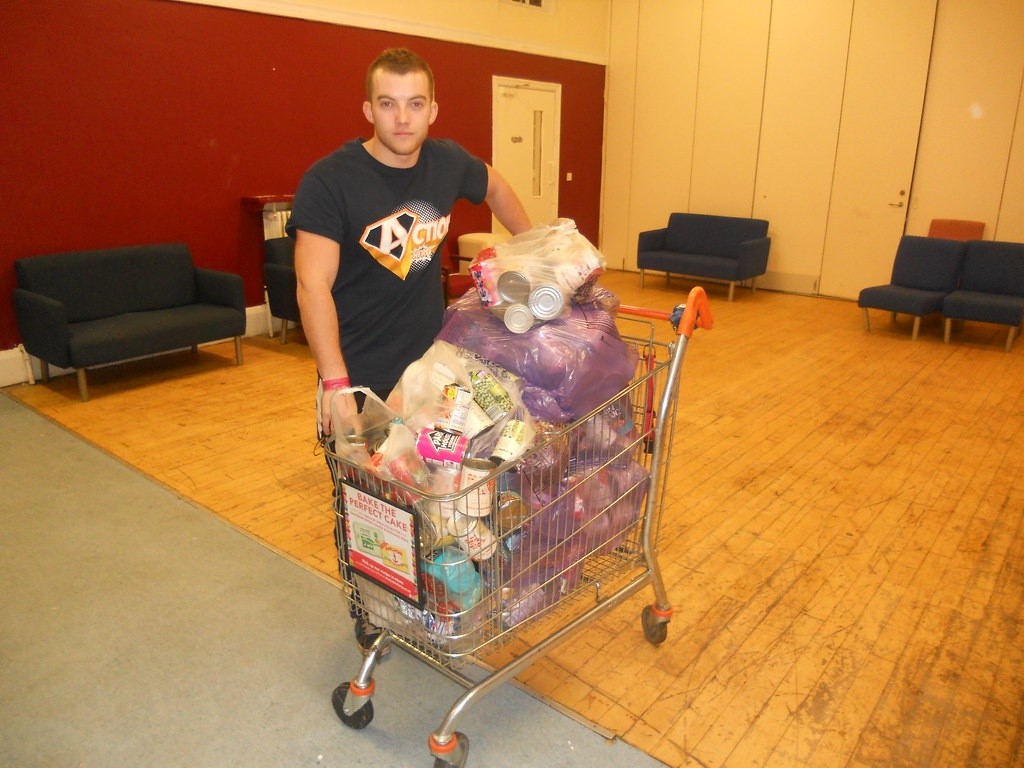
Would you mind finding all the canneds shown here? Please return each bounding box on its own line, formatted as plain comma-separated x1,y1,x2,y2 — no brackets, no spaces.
336,218,648,634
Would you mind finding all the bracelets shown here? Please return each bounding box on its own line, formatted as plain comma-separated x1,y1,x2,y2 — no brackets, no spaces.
322,377,350,390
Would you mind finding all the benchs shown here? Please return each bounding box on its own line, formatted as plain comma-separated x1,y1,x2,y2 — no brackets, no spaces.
9,241,246,403
638,212,771,301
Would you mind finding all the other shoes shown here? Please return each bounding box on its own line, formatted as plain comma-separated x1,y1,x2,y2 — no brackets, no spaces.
352,617,391,656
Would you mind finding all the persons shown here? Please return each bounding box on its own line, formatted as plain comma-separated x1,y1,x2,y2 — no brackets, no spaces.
284,50,532,656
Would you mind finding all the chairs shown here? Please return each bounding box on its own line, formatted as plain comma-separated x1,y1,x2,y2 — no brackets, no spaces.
928,219,986,241
440,243,473,308
942,240,1024,353
858,233,967,340
262,238,301,345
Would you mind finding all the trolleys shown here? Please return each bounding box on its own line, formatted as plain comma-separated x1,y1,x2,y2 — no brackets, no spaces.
311,286,716,768
